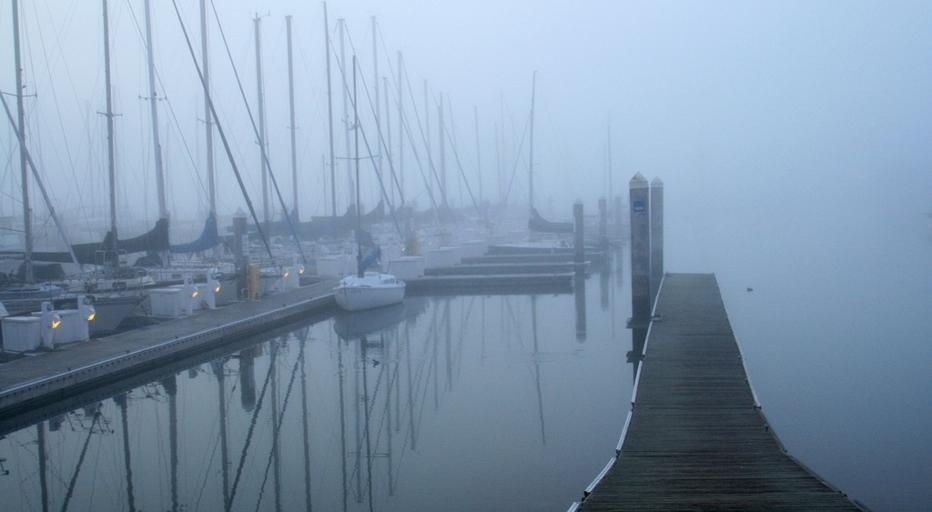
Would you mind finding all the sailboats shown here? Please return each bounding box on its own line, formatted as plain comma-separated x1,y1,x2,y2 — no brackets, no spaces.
0,0,619,357
